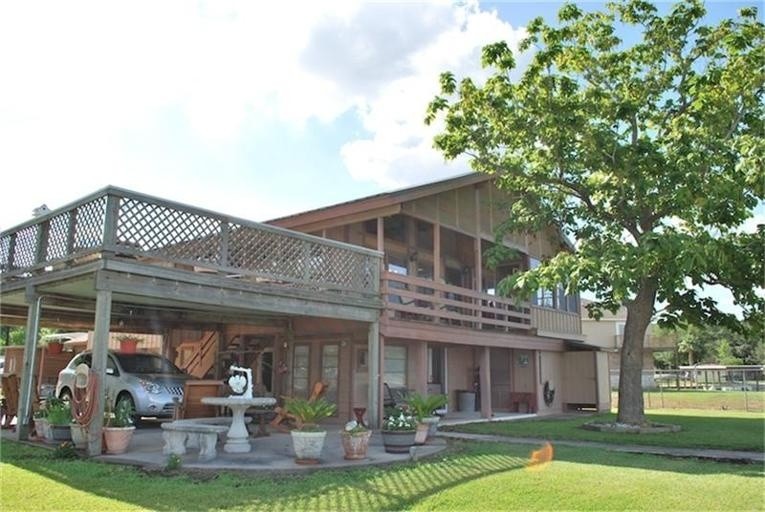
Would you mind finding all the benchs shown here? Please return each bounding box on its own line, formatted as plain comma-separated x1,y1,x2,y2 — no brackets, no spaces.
245,409,273,438
173,416,254,448
160,423,230,457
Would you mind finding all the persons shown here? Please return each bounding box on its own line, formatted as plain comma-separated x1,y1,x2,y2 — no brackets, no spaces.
472,367,481,410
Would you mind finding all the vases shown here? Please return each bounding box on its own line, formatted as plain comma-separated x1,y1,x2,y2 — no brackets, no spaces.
414,429,429,444
382,429,416,452
509,403,532,414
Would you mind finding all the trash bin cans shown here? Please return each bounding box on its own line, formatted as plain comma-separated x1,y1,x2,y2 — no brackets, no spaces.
457,390,476,412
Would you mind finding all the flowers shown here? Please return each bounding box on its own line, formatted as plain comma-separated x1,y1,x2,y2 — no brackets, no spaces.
382,411,419,430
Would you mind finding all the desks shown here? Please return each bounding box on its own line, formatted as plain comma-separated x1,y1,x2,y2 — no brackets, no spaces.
200,397,277,454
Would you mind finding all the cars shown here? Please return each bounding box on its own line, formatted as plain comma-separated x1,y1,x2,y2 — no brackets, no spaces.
55,348,198,426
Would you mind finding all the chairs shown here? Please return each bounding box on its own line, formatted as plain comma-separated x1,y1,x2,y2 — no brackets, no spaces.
270,380,329,434
383,382,395,408
0,372,40,429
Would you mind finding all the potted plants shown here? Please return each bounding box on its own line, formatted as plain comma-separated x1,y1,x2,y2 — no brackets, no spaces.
33,392,136,454
339,423,373,459
45,336,64,354
112,335,144,354
397,389,452,442
281,396,338,464
